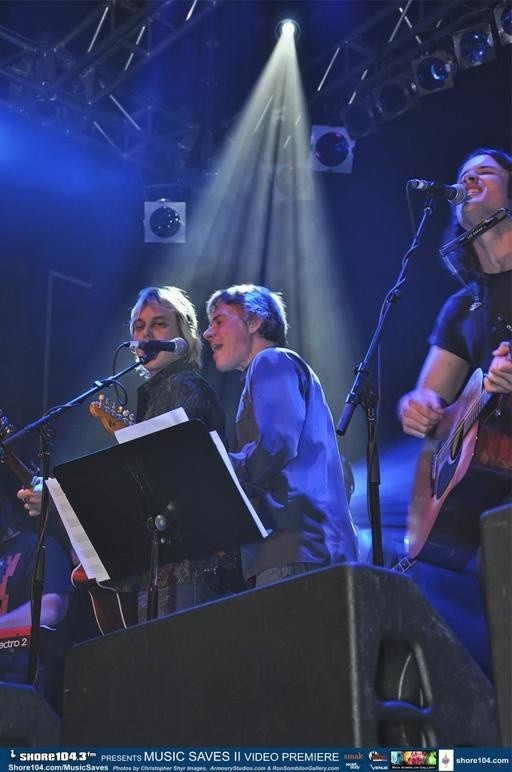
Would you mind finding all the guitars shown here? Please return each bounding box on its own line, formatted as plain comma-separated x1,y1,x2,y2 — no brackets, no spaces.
90,394,254,600
1,409,138,636
406,353,511,572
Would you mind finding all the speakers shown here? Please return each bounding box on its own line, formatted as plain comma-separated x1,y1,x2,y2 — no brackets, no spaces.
59,562,500,749
0,682,60,747
480,503,512,749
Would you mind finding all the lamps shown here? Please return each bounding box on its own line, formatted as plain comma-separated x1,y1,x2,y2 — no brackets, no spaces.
142,189,185,244
305,0,512,174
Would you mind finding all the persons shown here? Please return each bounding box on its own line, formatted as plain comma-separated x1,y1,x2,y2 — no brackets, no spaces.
0,481,70,747
394,149,512,684
202,281,360,588
17,286,252,623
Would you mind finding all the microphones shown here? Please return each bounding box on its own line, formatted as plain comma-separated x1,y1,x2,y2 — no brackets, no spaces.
124,337,189,356
409,179,466,205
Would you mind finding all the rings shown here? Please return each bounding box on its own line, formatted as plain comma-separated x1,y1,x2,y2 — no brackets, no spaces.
24,498,32,503
